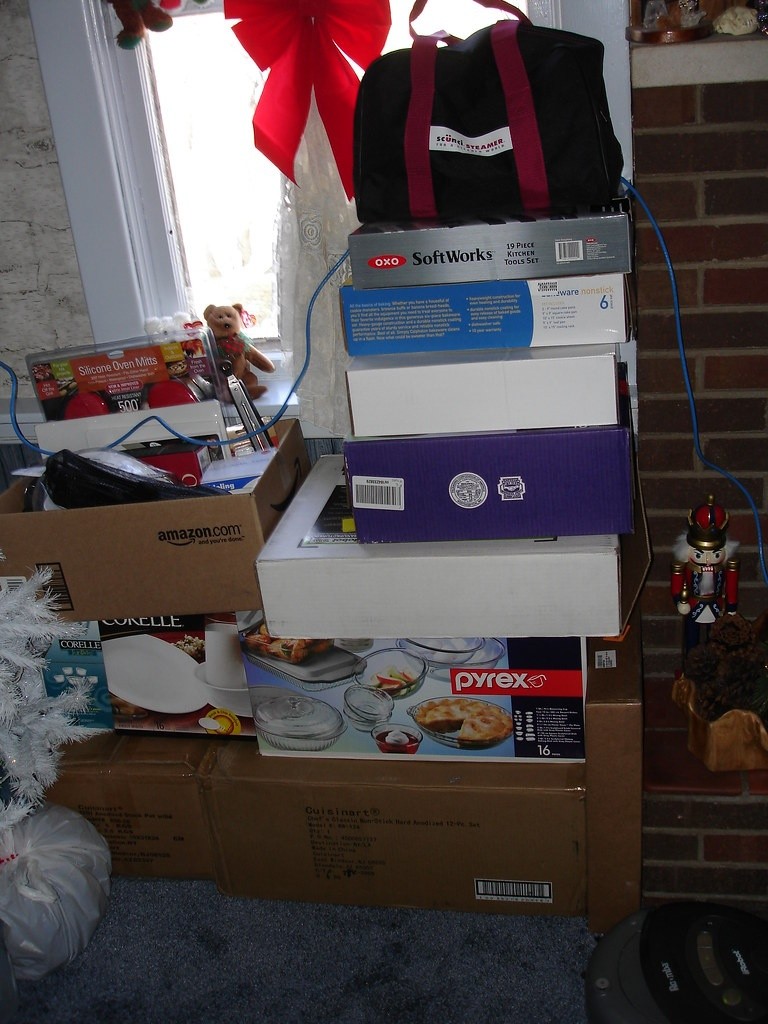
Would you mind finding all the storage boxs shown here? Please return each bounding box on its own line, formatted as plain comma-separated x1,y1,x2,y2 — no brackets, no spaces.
339,273,632,357
347,201,633,289
35,620,115,729
0,417,311,622
341,420,636,546
255,453,623,640
585,600,643,933
232,610,586,764
199,738,587,916
43,728,218,880
344,343,621,437
97,610,256,738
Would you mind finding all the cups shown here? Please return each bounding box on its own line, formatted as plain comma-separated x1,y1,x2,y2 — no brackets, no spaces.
207,624,244,688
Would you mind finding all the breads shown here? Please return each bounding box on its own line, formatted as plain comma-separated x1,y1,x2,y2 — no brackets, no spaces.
416,699,514,748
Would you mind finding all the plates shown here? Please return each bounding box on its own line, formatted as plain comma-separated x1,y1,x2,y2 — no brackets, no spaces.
196,663,248,692
102,633,208,714
407,696,513,749
207,688,252,717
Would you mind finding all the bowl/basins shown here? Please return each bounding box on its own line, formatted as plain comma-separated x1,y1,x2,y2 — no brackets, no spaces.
343,684,394,731
396,638,505,682
351,648,428,699
254,695,348,751
399,638,485,667
371,724,423,754
